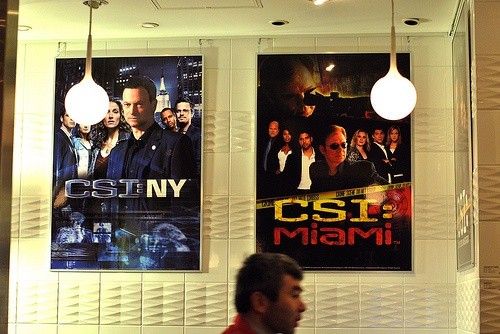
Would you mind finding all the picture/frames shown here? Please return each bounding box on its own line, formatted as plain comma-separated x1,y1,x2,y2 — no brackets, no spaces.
255,53,415,274
49,54,205,272
450,0,475,271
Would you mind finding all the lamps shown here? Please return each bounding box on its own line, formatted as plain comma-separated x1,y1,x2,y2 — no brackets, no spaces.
65,0,110,126
371,1,417,121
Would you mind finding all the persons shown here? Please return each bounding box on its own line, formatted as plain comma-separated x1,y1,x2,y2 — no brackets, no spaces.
253,54,375,118
347,128,411,183
54,75,201,188
257,121,325,198
309,125,390,195
221,253,307,334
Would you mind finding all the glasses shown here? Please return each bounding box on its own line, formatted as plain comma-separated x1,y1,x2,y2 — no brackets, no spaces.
326,143,348,150
